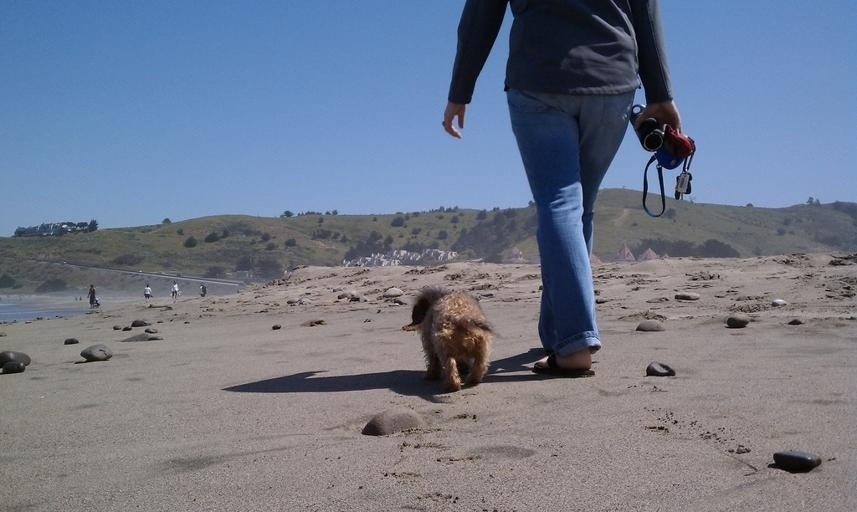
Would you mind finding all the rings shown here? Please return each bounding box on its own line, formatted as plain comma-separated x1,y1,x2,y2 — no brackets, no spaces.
442,121,445,126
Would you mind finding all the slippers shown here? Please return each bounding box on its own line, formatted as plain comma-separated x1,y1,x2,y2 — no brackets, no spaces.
531,353,596,378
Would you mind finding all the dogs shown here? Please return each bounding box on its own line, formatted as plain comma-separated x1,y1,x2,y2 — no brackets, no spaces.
402,285,495,392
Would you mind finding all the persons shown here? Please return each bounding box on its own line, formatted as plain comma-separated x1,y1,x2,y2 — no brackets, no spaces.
171,280,178,300
199,285,206,297
144,284,152,304
87,284,96,309
442,0,681,378
